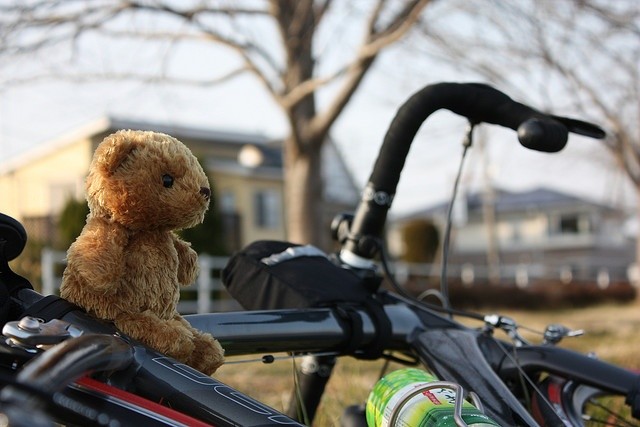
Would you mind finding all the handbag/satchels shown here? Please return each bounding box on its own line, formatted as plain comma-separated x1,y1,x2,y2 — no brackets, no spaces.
222,240,370,307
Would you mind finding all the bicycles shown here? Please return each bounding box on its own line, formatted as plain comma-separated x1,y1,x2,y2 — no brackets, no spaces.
2,81,639,426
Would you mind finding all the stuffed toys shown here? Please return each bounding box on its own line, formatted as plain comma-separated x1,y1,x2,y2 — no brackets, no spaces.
59,130,225,375
